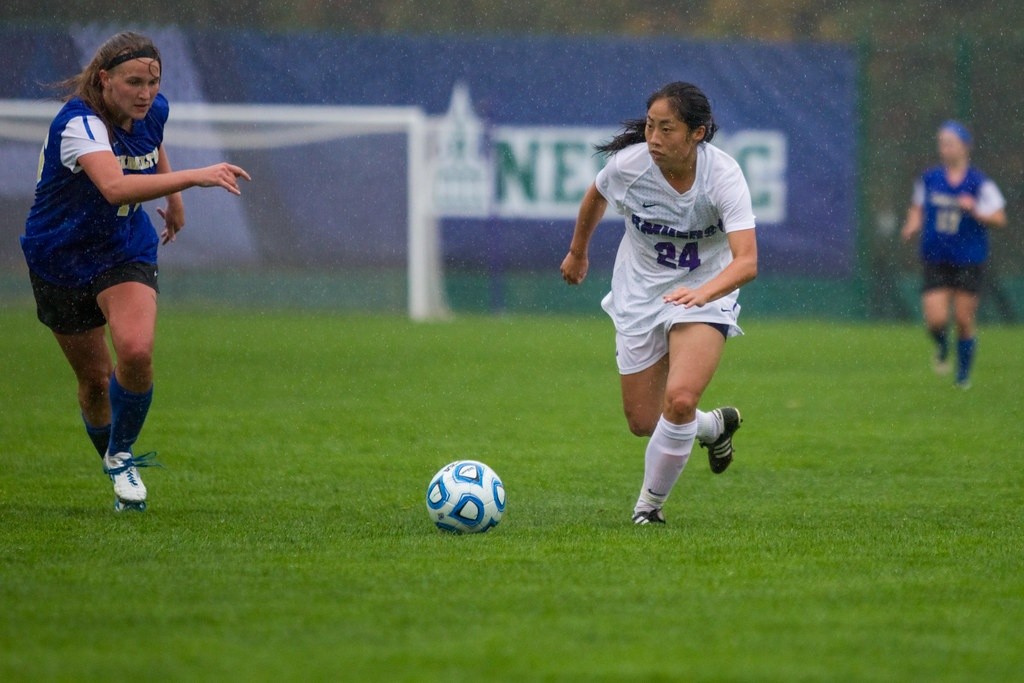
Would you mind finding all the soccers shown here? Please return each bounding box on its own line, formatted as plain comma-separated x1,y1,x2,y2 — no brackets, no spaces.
424,459,508,535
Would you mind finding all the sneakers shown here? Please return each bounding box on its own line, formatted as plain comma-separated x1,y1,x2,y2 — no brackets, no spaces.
631,508,666,526
698,407,744,475
102,446,163,513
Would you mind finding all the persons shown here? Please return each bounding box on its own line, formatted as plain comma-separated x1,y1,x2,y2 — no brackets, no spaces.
18,31,252,512
559,82,759,526
902,119,1008,391
